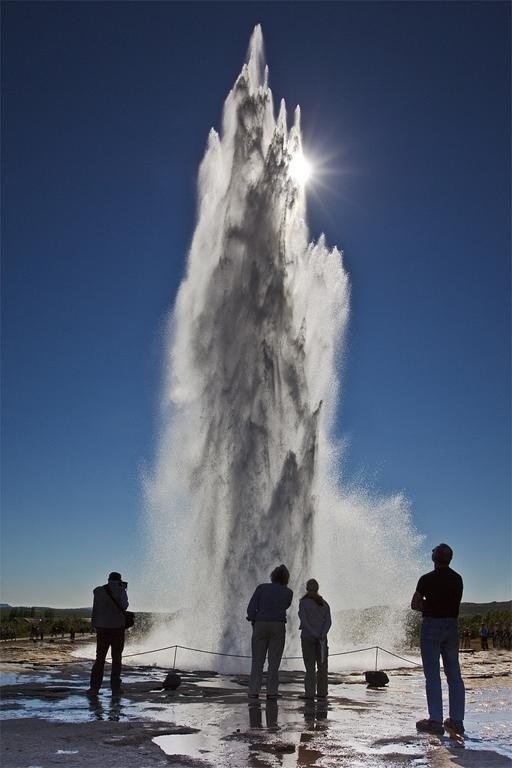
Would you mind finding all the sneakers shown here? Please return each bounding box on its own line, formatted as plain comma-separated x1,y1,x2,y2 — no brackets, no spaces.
87,688,124,695
444,718,464,732
299,694,327,698
248,694,282,698
416,720,444,734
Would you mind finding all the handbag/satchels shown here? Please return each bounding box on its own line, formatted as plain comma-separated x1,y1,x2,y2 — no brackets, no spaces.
123,611,135,628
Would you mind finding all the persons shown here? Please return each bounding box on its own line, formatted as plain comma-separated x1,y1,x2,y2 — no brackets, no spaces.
409,542,468,736
501,620,512,651
244,564,294,698
478,622,490,651
463,625,471,650
492,622,502,648
86,571,130,696
297,577,333,701
29,623,76,642
